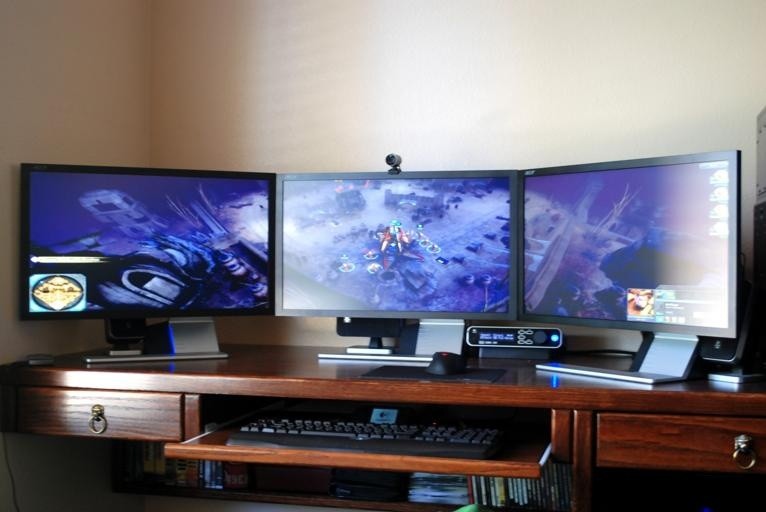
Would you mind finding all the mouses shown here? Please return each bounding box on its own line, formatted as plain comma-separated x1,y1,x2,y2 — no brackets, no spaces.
426,349,466,379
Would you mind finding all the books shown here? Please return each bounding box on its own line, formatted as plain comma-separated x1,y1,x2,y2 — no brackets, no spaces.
141,436,249,492
408,464,572,510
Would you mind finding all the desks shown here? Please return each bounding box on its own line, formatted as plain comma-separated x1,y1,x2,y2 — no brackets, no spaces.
0,343,766,512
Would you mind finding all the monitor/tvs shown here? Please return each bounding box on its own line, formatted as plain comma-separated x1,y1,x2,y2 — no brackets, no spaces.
519,148,740,386
276,169,520,366
19,164,275,363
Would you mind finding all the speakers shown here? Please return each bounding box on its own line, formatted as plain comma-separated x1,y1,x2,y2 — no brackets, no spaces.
701,280,762,385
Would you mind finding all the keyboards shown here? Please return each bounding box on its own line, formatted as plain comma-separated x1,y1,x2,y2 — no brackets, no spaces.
226,414,520,458
